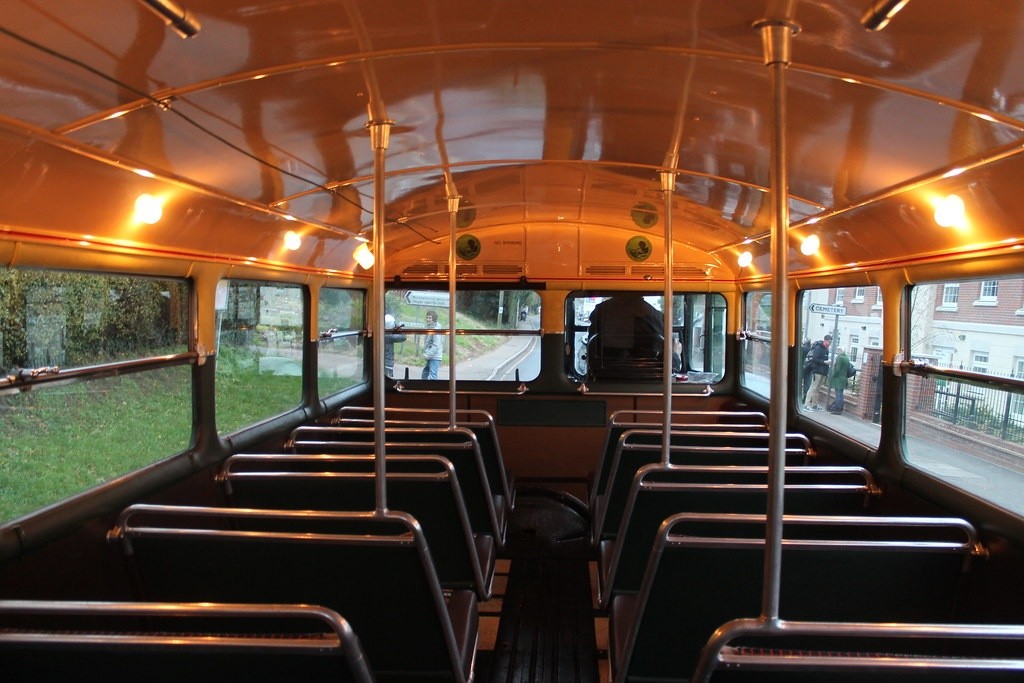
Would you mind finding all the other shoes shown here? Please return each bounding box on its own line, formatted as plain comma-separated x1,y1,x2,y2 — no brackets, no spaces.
830,411,841,415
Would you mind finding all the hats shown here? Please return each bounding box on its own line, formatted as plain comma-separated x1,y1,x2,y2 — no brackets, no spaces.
384,314,396,329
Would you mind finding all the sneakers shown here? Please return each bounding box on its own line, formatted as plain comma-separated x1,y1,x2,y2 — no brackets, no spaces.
810,405,825,411
802,405,814,413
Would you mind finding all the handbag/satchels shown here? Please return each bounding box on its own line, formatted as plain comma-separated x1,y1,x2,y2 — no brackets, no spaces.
847,363,856,378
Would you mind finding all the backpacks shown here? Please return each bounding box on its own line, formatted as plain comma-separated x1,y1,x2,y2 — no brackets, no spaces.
802,340,818,374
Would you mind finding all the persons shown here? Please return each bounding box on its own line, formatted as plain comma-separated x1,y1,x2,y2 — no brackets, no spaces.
588,291,681,375
422,312,443,379
521,302,528,320
384,315,406,378
801,335,855,415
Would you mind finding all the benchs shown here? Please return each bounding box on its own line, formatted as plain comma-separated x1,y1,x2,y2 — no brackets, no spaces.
0,407,1024,683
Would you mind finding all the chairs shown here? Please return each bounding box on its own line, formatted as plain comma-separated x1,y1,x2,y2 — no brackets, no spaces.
586,332,681,386
